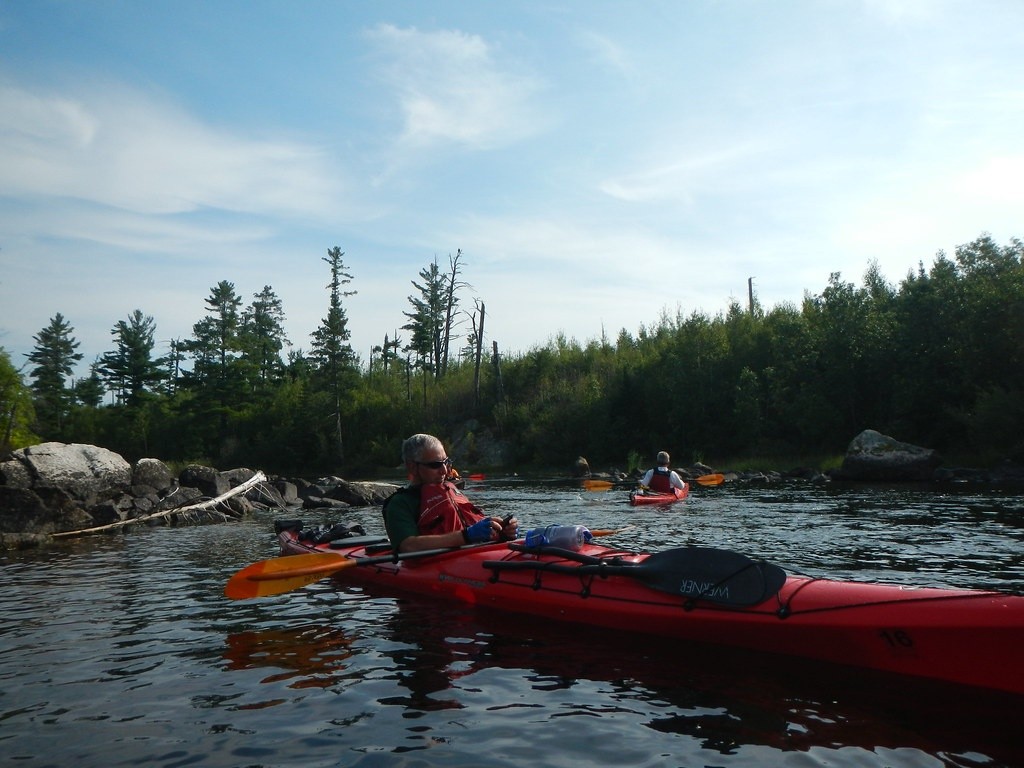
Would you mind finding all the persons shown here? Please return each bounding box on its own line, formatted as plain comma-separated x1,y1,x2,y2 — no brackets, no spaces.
640,451,686,494
445,458,461,481
382,434,519,554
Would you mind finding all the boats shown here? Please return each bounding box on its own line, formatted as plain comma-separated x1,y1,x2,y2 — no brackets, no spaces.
265,515,1023,694
451,480,465,490
631,482,689,507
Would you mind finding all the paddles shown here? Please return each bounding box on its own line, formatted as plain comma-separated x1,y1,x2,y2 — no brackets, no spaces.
225,525,631,599
408,473,482,481
584,474,724,491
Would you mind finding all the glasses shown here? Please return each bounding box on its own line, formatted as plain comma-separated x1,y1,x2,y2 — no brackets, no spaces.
414,459,454,469
